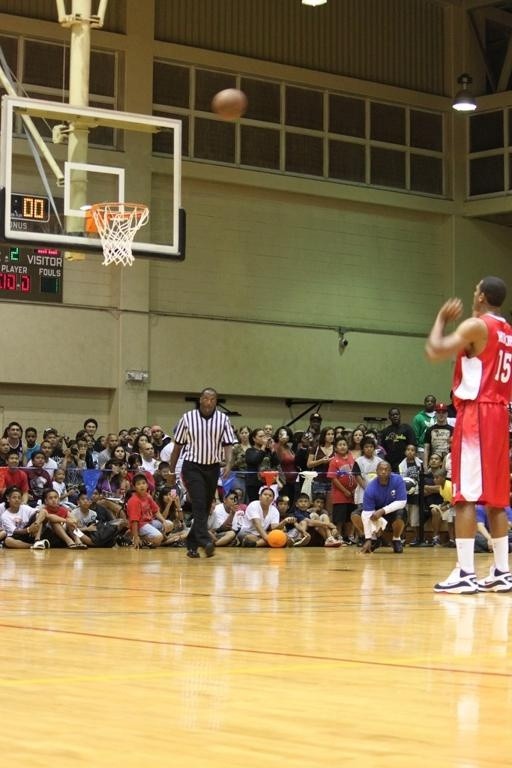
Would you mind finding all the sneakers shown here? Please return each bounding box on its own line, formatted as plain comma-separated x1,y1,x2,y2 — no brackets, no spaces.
287,534,312,547
241,535,257,548
324,536,341,547
367,540,378,552
432,565,477,594
391,539,402,552
32,534,188,547
476,565,512,592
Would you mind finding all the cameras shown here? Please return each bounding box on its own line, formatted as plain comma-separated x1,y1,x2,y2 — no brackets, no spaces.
71,447,79,455
266,435,272,441
303,432,313,439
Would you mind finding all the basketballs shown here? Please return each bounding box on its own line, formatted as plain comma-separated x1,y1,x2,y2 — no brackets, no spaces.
214,88,246,119
268,529,286,546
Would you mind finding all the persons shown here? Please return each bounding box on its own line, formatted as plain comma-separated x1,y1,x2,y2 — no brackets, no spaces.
422,274,512,596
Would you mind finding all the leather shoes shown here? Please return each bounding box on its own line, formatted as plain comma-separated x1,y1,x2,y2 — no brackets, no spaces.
185,537,201,558
204,541,215,557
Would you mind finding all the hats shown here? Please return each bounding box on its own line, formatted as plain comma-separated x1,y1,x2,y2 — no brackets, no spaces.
151,426,164,436
309,413,321,420
433,402,447,412
